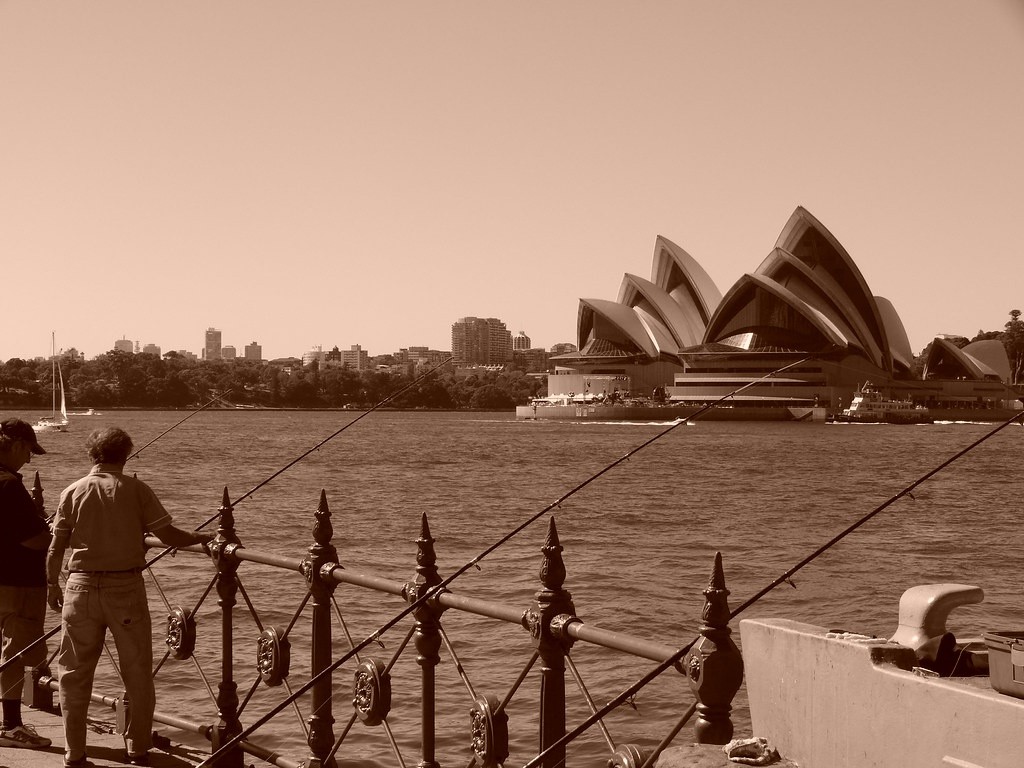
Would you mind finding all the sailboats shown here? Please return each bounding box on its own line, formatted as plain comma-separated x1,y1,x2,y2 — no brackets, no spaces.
28,331,70,434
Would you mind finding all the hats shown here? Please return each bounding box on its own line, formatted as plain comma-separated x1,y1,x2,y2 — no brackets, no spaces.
0,418,47,455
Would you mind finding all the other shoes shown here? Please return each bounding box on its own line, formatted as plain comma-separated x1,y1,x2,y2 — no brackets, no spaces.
122,750,149,765
64,751,86,768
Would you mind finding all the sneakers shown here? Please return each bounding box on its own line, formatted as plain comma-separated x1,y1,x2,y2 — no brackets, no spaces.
0,724,52,748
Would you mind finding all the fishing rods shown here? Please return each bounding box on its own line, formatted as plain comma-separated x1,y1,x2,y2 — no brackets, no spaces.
44,385,235,522
196,352,818,768
0,355,456,673
521,409,1024,768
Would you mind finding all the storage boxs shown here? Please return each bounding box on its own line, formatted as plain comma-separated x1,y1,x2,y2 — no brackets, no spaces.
979,631,1024,701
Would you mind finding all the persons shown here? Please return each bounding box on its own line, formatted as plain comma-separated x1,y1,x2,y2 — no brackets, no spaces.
46,424,217,768
0,418,52,749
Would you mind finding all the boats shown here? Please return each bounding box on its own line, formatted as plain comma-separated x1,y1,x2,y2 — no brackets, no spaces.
824,378,930,424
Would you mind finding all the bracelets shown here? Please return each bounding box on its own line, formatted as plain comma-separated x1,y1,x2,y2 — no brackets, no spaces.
47,582,59,587
